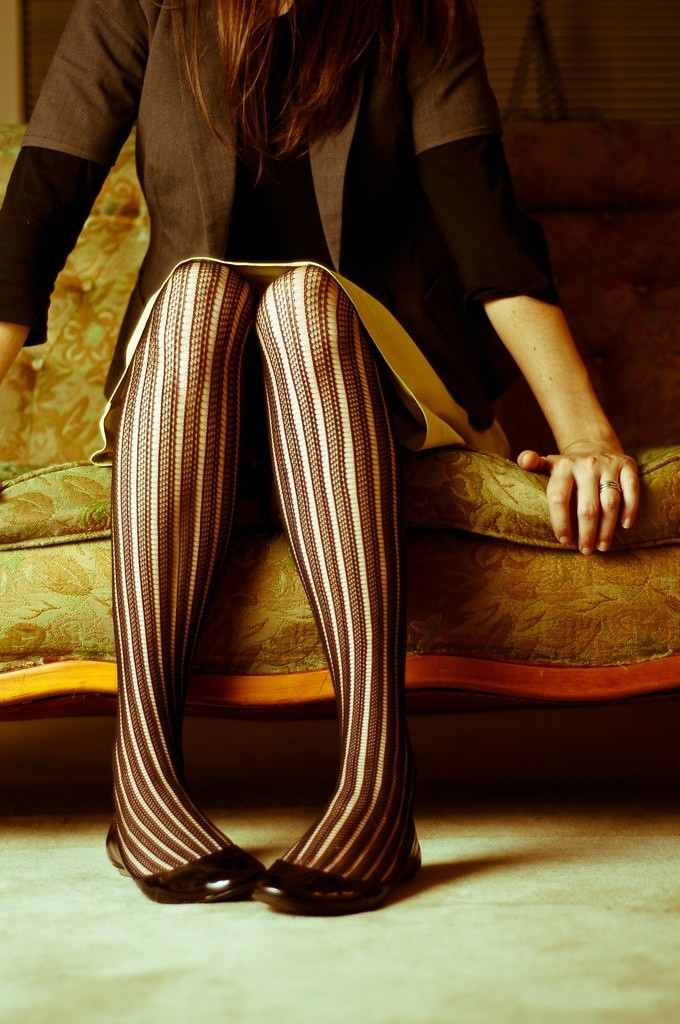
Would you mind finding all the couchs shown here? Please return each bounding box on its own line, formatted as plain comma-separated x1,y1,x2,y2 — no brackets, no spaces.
0,112,680,723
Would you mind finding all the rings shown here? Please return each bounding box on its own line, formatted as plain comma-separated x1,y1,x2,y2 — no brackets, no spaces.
599,480,621,492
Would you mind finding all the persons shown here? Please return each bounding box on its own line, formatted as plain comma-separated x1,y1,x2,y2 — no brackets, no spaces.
0,0,639,916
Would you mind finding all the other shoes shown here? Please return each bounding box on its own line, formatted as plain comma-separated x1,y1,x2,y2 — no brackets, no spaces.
107,812,266,904
254,814,422,916
